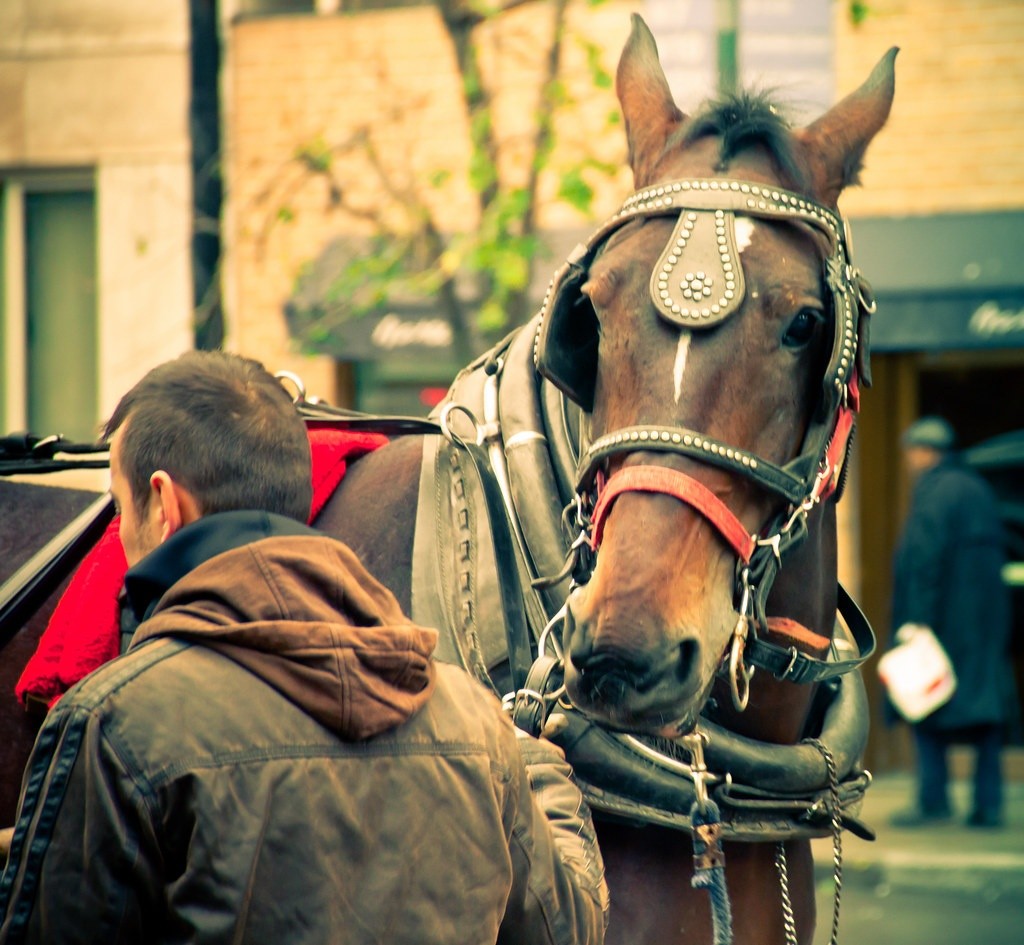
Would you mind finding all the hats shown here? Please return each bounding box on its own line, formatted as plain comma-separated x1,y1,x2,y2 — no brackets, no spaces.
901,418,957,451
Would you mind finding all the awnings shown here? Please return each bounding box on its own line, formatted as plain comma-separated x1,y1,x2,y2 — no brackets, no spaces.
846,207,1022,358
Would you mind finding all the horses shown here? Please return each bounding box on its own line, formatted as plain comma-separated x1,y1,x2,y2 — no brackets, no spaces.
2,10,899,945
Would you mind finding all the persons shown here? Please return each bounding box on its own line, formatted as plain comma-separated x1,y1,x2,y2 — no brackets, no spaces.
1,351,618,945
879,423,1021,831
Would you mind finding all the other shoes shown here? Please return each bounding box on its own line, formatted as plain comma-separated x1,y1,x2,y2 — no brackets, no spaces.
891,808,950,828
964,810,1000,828
